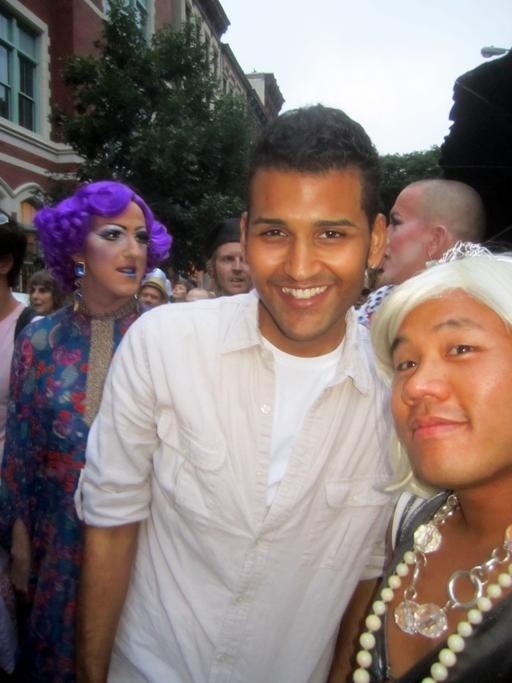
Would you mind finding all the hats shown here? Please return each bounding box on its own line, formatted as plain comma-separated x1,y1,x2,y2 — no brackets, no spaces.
142,268,171,304
207,217,239,259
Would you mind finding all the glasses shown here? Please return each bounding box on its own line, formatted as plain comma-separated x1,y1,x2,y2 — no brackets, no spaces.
0,212,13,226
